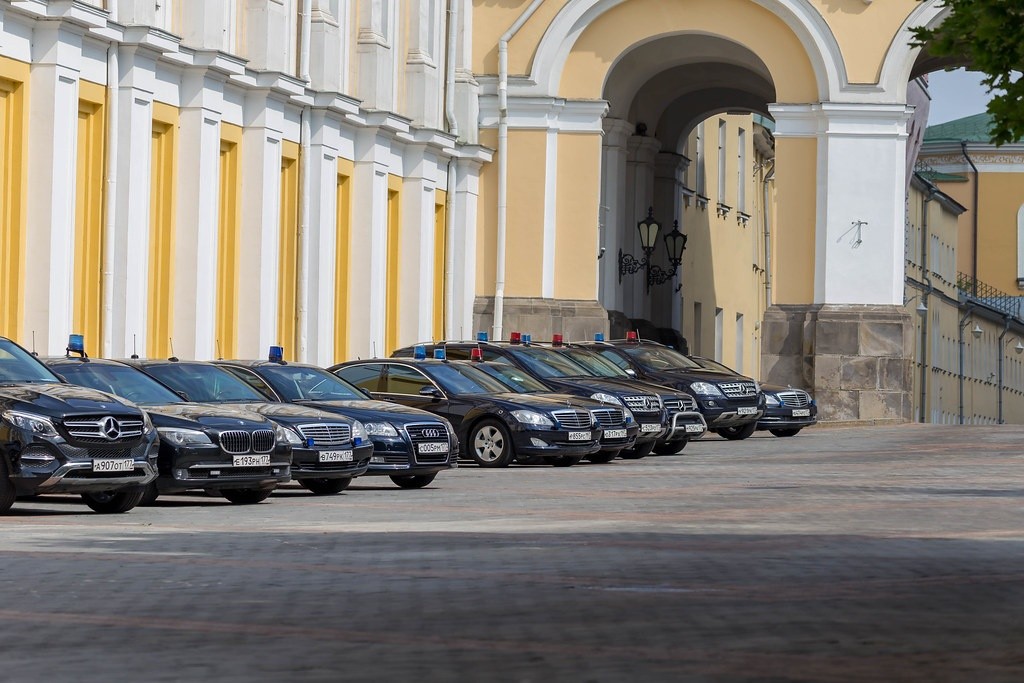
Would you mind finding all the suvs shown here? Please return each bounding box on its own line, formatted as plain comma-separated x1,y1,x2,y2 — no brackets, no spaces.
0,336,161,513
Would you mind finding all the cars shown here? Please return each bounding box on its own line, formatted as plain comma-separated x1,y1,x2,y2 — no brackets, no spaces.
34,331,819,505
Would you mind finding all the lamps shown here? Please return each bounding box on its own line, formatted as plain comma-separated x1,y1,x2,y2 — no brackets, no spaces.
962,321,983,338
618,206,666,284
1006,336,1024,354
904,295,928,317
646,220,688,295
